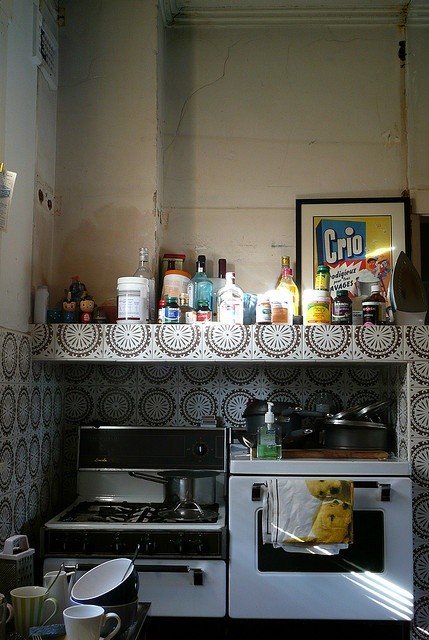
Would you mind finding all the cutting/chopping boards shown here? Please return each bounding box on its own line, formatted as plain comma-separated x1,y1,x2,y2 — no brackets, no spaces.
250,448,389,459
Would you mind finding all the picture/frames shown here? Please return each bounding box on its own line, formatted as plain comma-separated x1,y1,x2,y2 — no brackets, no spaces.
295,192,410,312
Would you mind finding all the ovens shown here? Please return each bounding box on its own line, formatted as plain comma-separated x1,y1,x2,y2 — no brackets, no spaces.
226,476,414,624
41,532,227,616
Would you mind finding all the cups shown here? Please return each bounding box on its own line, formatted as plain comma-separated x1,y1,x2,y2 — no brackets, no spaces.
11,586,59,635
63,604,121,640
42,571,75,624
0,593,14,640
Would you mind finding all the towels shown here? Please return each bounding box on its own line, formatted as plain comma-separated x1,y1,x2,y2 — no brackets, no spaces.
262,479,354,556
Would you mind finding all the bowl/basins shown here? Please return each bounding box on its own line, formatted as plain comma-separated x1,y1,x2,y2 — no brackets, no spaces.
69,597,141,639
71,558,139,605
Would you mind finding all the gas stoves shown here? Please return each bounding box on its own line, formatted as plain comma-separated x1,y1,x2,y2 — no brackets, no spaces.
52,500,221,522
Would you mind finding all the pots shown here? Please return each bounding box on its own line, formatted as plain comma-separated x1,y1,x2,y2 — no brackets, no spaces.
322,399,394,451
242,400,335,419
128,471,221,506
244,415,313,443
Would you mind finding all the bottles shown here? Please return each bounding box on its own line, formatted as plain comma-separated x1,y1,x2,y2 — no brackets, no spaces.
188,262,212,309
157,300,166,324
351,297,364,325
276,255,295,288
304,289,331,324
315,266,330,290
270,293,293,325
275,268,302,323
116,276,150,324
217,271,243,324
161,296,180,324
331,290,353,324
361,298,380,325
196,300,212,322
132,248,155,322
177,292,197,324
32,284,50,324
255,296,272,325
69,276,87,322
161,269,192,297
370,286,387,325
